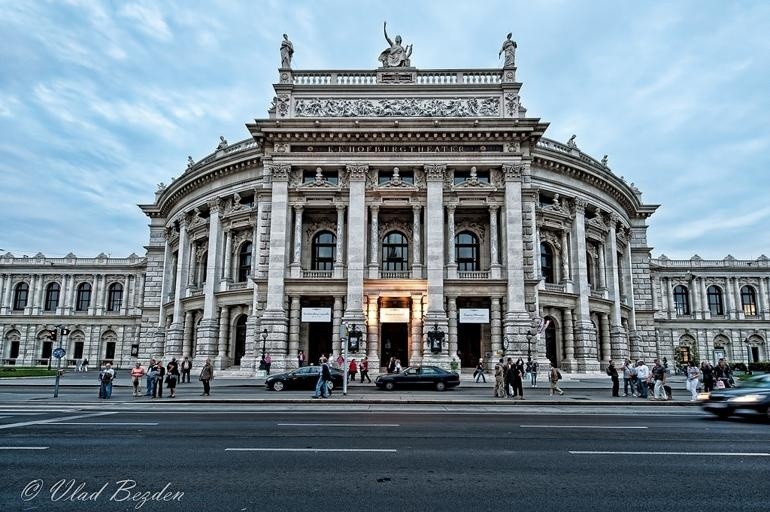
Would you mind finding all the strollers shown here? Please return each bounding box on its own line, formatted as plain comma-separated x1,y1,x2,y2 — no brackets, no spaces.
716,375,736,389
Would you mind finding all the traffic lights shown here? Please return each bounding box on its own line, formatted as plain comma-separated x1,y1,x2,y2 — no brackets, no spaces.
62,329,70,335
46,329,57,341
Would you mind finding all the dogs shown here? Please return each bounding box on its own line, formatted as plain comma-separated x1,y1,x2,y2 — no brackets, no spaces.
648,383,672,399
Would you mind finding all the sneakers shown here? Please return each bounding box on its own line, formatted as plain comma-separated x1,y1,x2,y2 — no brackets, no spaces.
200,393,209,396
494,394,525,400
152,395,162,398
611,393,668,401
311,394,327,399
166,395,175,398
144,394,151,396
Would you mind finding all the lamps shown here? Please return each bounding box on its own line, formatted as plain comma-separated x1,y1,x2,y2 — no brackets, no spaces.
427,323,447,355
345,323,363,354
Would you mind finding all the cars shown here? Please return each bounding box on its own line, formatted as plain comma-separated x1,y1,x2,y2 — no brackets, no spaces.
374,366,459,394
265,364,351,391
697,372,770,420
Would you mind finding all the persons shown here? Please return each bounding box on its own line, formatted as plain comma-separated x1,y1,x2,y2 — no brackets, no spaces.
298,351,306,367
393,355,403,373
386,356,394,373
198,357,214,397
493,356,539,399
188,154,193,165
264,352,272,375
101,362,115,399
217,134,227,148
602,154,607,166
475,357,487,383
130,356,192,399
451,357,457,371
499,32,518,67
685,358,735,400
312,352,372,399
382,21,414,66
604,357,670,401
569,133,575,148
82,356,89,372
546,362,563,395
279,33,293,66
74,358,82,373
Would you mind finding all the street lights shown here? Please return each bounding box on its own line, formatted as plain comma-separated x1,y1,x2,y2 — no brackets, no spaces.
525,330,534,373
744,335,752,376
260,328,269,365
